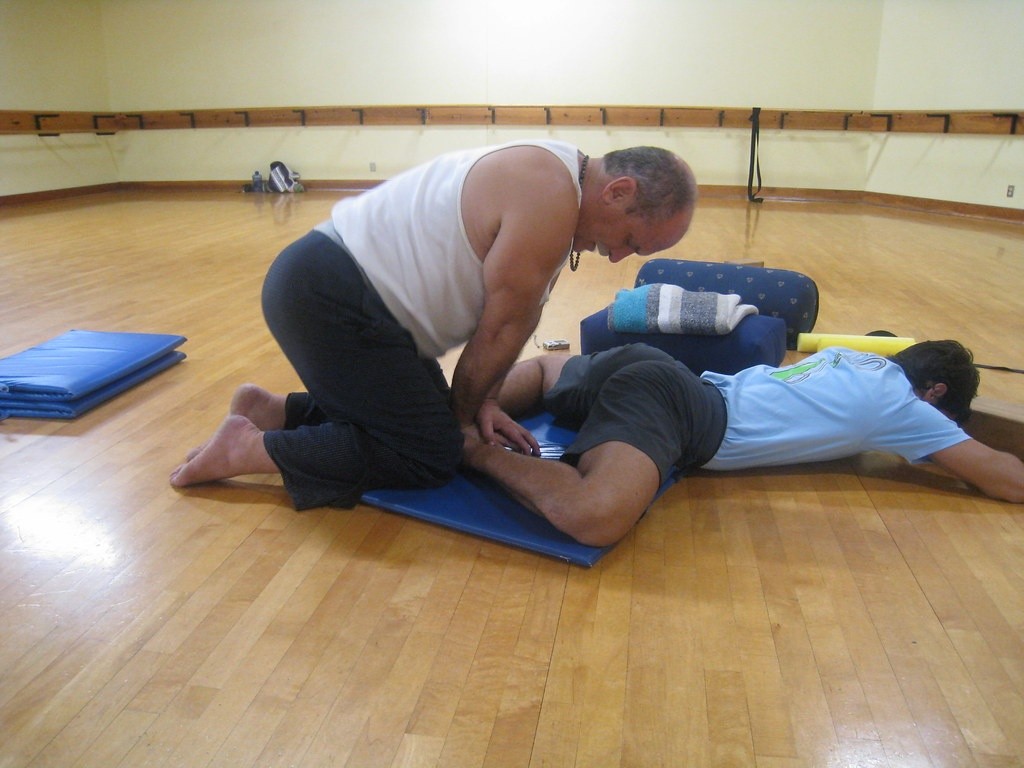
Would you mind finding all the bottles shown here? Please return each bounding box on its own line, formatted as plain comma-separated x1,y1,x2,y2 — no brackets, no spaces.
251,170,263,192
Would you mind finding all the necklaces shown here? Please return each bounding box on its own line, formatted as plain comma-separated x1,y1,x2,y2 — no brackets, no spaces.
569,155,589,271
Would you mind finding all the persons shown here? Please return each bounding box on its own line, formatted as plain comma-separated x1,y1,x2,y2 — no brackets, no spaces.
460,338,1024,547
169,137,697,512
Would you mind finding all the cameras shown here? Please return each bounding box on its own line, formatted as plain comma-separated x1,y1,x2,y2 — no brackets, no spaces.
543,339,570,350
